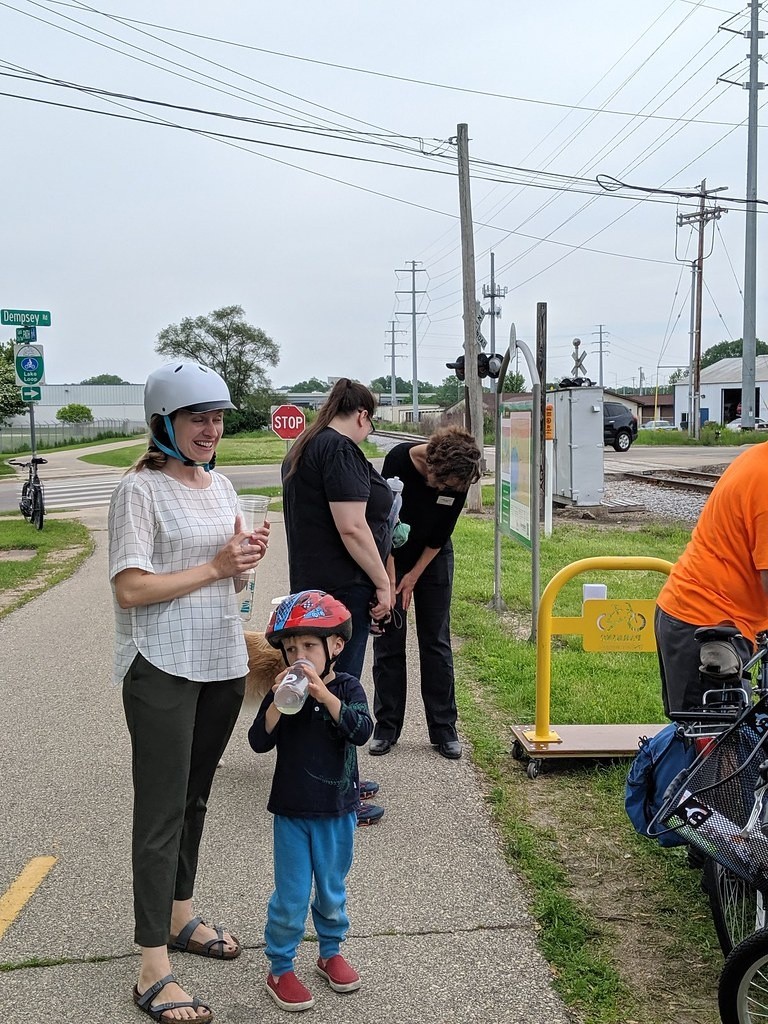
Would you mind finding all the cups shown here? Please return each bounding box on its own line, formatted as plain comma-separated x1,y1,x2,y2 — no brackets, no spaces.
697,737,717,766
236,494,271,535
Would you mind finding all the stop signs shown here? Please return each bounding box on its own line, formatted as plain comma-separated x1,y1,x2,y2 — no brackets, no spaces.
271,405,306,441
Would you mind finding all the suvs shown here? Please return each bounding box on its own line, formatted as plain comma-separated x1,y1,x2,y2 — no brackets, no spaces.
603,402,638,452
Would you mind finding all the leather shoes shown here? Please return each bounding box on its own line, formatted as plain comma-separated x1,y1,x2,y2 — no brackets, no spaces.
369,738,397,756
440,741,463,760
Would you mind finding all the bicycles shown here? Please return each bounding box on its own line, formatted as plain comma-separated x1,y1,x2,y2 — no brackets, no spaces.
646,625,768,1024
8,457,48,531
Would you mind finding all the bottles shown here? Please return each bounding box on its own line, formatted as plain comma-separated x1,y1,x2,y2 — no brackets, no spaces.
387,475,404,530
274,660,316,715
224,539,256,622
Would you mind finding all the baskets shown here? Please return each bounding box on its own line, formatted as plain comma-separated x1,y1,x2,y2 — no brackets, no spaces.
646,690,768,895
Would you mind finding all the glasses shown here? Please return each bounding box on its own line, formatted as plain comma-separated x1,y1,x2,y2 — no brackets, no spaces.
357,409,376,435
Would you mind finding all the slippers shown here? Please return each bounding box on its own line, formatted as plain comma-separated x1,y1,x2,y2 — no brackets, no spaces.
169,916,241,961
133,973,213,1024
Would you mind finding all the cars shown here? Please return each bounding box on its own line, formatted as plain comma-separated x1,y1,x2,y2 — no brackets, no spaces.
639,421,678,433
725,418,768,433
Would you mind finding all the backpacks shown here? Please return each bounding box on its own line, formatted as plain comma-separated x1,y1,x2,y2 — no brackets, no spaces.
625,722,707,847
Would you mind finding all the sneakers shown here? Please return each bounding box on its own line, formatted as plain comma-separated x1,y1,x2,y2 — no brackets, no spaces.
359,780,380,800
265,970,315,1012
356,800,385,827
314,954,362,993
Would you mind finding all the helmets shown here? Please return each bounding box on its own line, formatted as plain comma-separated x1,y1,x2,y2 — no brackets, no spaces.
143,361,238,429
263,589,353,651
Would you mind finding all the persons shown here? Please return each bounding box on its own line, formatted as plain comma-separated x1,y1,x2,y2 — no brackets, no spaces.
369,425,484,760
654,439,768,895
110,359,272,1024
248,590,375,1012
281,379,394,827
